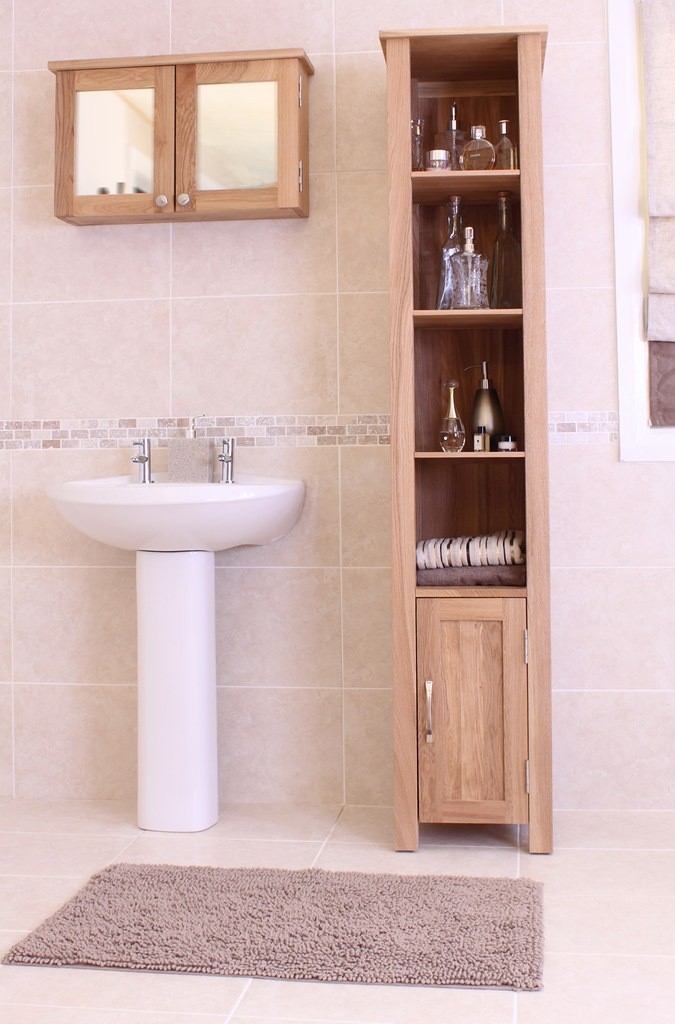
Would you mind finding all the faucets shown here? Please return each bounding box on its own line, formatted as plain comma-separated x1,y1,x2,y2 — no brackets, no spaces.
129,437,155,484
216,437,234,485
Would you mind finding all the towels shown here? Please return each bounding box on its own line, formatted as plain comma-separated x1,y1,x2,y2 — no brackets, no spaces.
415,565,526,588
415,530,524,570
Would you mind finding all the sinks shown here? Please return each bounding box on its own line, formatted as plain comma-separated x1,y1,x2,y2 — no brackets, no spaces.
50,476,308,551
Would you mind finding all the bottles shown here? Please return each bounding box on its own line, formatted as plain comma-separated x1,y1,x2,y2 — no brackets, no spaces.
436,194,470,310
489,191,521,309
438,381,467,453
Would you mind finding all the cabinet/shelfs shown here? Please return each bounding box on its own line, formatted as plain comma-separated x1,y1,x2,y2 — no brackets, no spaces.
378,25,548,856
46,47,314,226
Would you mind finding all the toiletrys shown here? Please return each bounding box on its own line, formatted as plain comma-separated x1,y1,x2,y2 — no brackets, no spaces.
165,415,214,484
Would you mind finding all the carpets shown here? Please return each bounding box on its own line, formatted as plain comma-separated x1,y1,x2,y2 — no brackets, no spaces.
0,862,542,990
